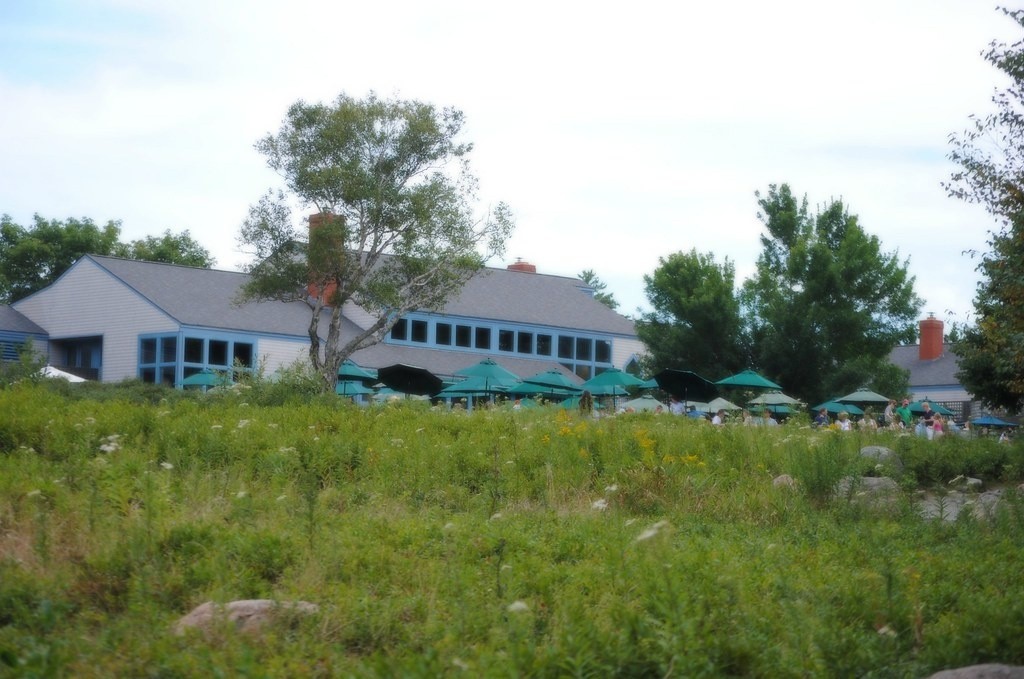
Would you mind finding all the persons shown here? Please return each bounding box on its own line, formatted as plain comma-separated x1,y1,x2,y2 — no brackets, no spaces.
670,396,710,420
999,427,1015,447
883,400,983,440
579,390,634,419
654,405,664,416
712,409,726,426
814,408,886,434
743,409,778,426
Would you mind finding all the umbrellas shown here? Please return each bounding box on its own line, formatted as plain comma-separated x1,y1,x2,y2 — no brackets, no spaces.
813,386,893,415
956,416,1019,429
333,358,804,415
9,364,88,385
896,396,957,416
171,368,319,394
